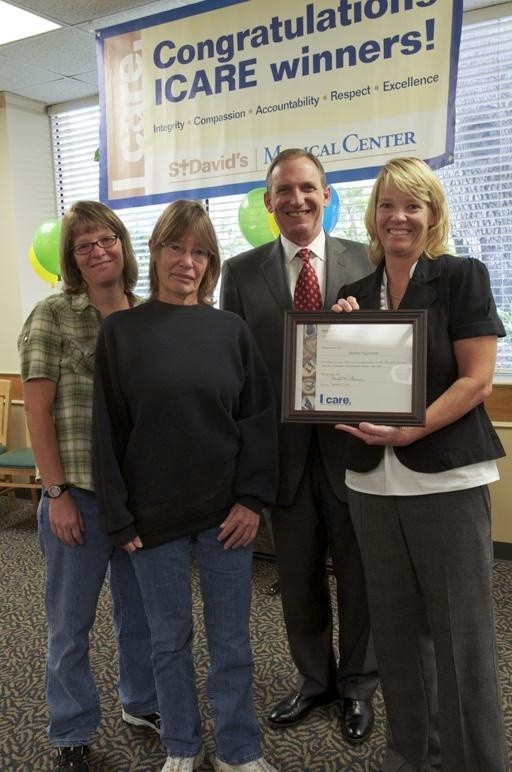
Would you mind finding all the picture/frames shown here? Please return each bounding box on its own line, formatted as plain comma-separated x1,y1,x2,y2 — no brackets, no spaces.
282,310,427,427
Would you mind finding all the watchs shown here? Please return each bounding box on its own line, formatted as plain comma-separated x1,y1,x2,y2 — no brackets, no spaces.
44,484,67,497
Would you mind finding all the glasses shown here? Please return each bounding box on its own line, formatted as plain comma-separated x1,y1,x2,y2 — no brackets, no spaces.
70,234,120,255
159,240,212,261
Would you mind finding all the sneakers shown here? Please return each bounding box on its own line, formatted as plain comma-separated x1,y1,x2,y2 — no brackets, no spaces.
52,742,91,772
214,754,277,771
120,704,164,736
159,741,206,772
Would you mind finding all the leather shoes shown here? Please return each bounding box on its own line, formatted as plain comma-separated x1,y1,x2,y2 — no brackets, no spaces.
339,699,375,744
266,686,340,729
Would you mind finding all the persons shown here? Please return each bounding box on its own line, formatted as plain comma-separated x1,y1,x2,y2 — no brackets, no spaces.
332,158,510,772
221,150,386,745
19,201,163,771
92,200,282,772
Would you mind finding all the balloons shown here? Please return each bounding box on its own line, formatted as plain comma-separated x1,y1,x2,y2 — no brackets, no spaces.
32,219,62,280
269,213,281,237
30,246,58,289
240,186,275,246
323,183,340,234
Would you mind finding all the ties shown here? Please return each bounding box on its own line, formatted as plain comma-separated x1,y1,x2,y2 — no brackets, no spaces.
293,248,324,313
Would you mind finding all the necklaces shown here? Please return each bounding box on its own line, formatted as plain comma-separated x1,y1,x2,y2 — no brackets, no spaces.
387,292,404,300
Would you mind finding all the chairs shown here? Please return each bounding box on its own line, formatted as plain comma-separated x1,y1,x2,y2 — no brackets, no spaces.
0,378,13,456
0,405,42,526
